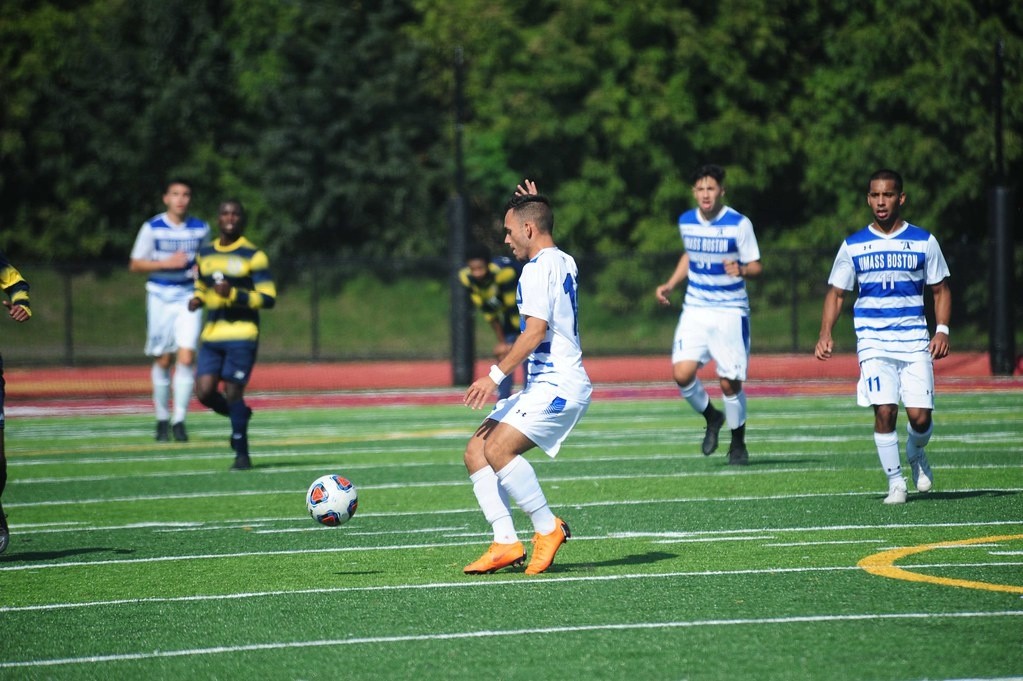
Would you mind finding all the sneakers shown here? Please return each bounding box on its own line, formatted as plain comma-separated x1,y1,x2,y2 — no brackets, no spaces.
464,540,527,575
883,477,908,503
726,441,749,465
525,517,570,574
906,439,934,492
702,411,724,455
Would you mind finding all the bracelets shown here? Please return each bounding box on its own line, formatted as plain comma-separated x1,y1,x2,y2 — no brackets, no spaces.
936,324,949,335
488,364,506,384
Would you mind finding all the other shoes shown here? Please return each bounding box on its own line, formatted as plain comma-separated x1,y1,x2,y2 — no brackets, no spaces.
173,431,188,441
0,502,9,553
230,455,252,470
156,432,170,442
231,408,252,448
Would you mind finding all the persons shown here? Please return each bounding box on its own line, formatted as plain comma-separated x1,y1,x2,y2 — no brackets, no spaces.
0,244,30,551
187,199,277,473
461,180,594,576
129,180,211,441
814,168,952,506
458,244,529,400
656,167,765,465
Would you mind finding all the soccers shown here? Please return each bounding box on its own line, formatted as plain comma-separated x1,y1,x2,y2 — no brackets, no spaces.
305,474,358,526
